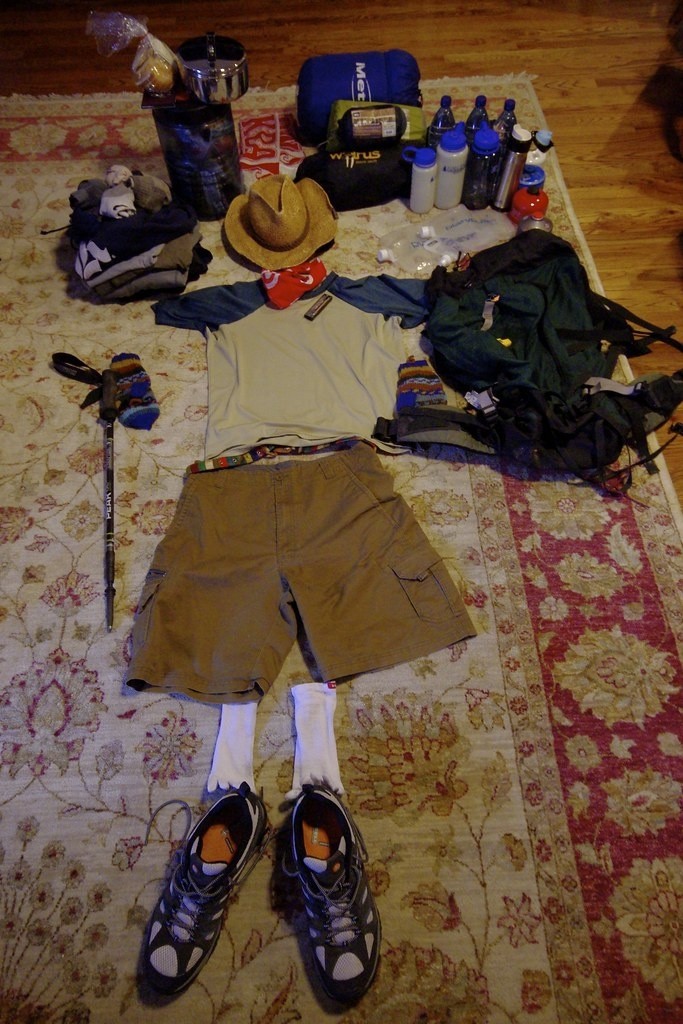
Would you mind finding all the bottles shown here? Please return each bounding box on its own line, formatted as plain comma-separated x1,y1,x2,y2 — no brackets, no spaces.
403,93,554,236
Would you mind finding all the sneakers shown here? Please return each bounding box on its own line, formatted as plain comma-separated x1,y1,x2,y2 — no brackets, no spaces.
281,784,380,1001
140,782,270,998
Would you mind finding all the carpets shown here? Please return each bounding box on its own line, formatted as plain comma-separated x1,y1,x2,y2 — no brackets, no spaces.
0,72,683,1024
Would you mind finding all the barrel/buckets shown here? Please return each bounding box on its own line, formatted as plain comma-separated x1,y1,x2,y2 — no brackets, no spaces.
151,103,245,223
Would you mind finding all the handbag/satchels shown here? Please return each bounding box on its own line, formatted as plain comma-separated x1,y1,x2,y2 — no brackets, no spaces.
296,49,423,147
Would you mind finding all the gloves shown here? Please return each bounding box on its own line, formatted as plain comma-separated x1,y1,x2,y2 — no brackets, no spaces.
396,359,445,411
108,352,161,432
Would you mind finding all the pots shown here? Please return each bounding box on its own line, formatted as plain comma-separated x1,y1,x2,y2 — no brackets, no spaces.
176,29,249,105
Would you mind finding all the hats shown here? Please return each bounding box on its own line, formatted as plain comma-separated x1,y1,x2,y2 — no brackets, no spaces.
225,174,339,270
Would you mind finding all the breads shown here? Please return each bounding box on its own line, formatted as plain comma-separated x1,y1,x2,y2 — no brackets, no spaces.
134,41,185,92
